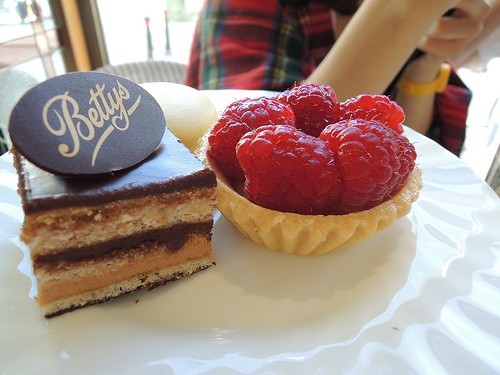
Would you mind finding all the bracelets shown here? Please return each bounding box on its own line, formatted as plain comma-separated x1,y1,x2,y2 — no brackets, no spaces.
397,62,451,97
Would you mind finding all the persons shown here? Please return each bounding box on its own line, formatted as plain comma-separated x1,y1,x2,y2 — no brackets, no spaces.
184,0,499,160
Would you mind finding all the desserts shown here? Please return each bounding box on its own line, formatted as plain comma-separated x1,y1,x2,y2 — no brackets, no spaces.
13,71,422,320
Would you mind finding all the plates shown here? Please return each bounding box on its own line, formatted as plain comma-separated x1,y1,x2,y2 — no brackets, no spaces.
0,89,500,375
